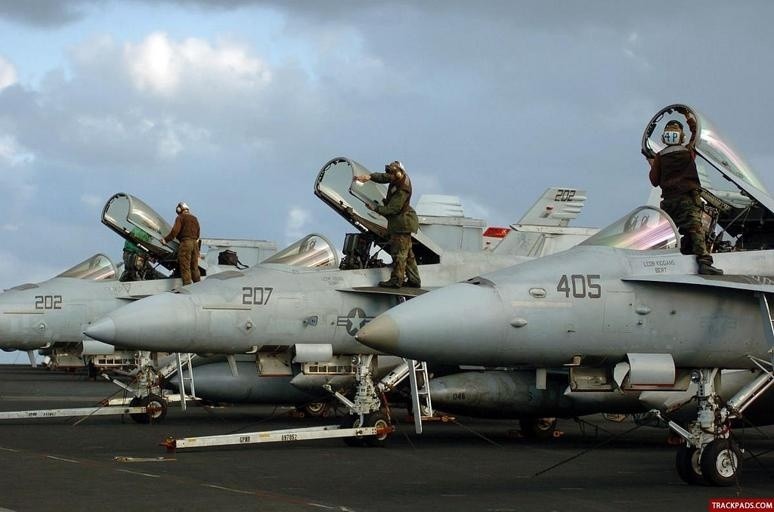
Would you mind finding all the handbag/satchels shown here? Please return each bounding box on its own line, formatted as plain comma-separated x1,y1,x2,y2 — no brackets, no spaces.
219,250,238,265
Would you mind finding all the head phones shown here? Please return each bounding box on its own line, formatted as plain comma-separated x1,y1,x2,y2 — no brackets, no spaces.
389,162,402,179
176,206,182,214
662,120,686,143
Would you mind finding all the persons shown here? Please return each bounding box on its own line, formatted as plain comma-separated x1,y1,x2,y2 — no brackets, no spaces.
648,120,723,276
355,159,421,289
123,220,157,280
162,201,200,286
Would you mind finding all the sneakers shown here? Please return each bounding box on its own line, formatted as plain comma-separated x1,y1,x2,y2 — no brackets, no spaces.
402,282,420,288
699,264,723,275
378,280,400,289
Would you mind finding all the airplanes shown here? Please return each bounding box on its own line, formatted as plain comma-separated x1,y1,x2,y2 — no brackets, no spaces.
78,153,598,455
0,193,280,381
351,103,774,486
